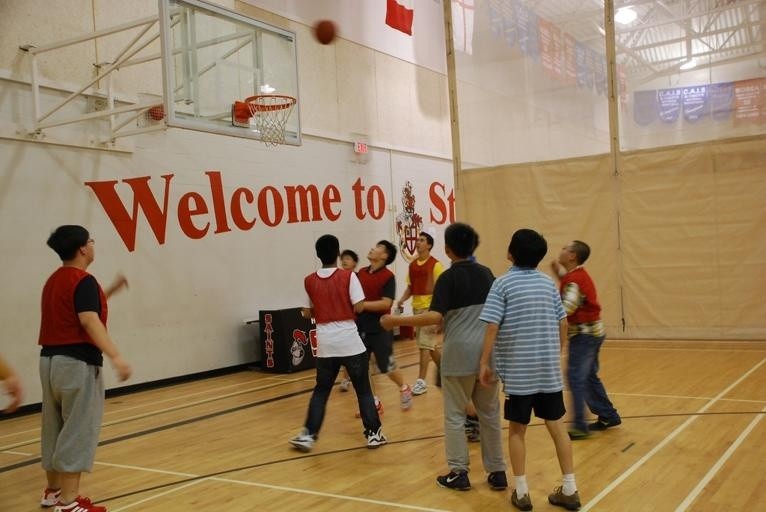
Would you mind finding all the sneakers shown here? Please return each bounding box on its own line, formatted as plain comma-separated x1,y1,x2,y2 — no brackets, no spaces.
437,471,471,490
412,378,428,394
42,487,62,506
54,495,106,510
399,384,412,409
354,401,384,417
488,470,508,489
567,428,592,440
589,416,621,431
511,489,533,511
548,485,580,510
464,414,482,441
288,427,318,452
340,378,350,391
364,426,387,449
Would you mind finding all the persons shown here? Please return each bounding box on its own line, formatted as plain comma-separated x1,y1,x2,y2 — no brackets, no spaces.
353,238,411,419
432,361,481,442
287,233,388,449
397,231,445,396
381,224,508,492
476,228,582,511
550,239,621,441
338,249,359,392
0,360,26,417
37,224,132,511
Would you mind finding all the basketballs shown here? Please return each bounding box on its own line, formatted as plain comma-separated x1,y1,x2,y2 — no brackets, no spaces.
314,21,337,45
